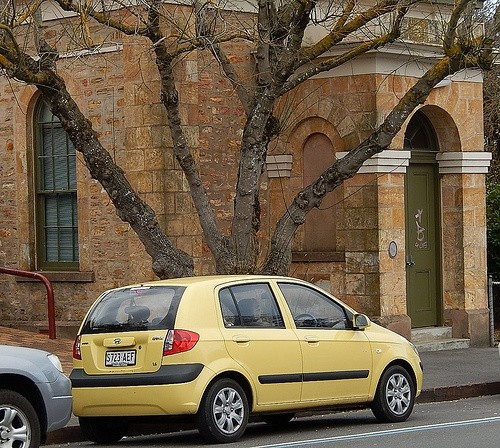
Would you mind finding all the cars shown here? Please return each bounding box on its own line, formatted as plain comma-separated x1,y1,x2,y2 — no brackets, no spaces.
68,272,425,445
0,344,75,448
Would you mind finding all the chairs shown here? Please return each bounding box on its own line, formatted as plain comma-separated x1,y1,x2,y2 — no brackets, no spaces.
233,299,272,326
125,305,153,327
